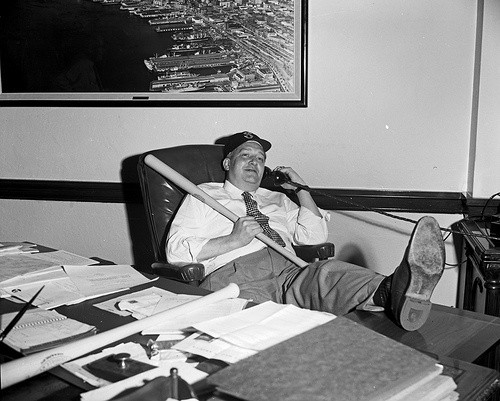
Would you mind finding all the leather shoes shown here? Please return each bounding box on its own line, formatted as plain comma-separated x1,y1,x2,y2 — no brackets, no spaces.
391,216,446,331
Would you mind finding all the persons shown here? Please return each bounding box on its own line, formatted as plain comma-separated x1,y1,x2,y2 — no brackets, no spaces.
165,131,446,331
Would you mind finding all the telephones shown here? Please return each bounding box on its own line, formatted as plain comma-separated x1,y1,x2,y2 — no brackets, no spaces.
264,167,500,243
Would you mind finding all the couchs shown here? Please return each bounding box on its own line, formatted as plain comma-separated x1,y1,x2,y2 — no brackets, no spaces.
121,143,335,342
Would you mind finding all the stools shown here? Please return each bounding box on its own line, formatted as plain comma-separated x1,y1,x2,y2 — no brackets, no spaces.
339,302,500,368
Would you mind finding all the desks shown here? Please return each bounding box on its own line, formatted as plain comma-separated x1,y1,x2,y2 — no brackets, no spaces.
0,241,500,401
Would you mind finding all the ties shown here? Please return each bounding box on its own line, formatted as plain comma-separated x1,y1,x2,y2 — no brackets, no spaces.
241,192,287,248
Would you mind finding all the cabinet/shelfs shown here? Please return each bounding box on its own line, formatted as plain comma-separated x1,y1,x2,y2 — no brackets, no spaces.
455,220,500,371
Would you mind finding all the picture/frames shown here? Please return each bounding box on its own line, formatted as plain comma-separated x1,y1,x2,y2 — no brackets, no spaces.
1,1,306,106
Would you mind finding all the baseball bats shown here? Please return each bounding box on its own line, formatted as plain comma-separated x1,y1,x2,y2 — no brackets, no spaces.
143,154,310,270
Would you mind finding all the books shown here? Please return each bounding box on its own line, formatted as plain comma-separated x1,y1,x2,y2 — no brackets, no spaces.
206,315,461,401
1,309,99,354
0,241,160,311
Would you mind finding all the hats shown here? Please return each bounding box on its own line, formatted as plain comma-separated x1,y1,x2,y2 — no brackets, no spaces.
223,131,273,159
